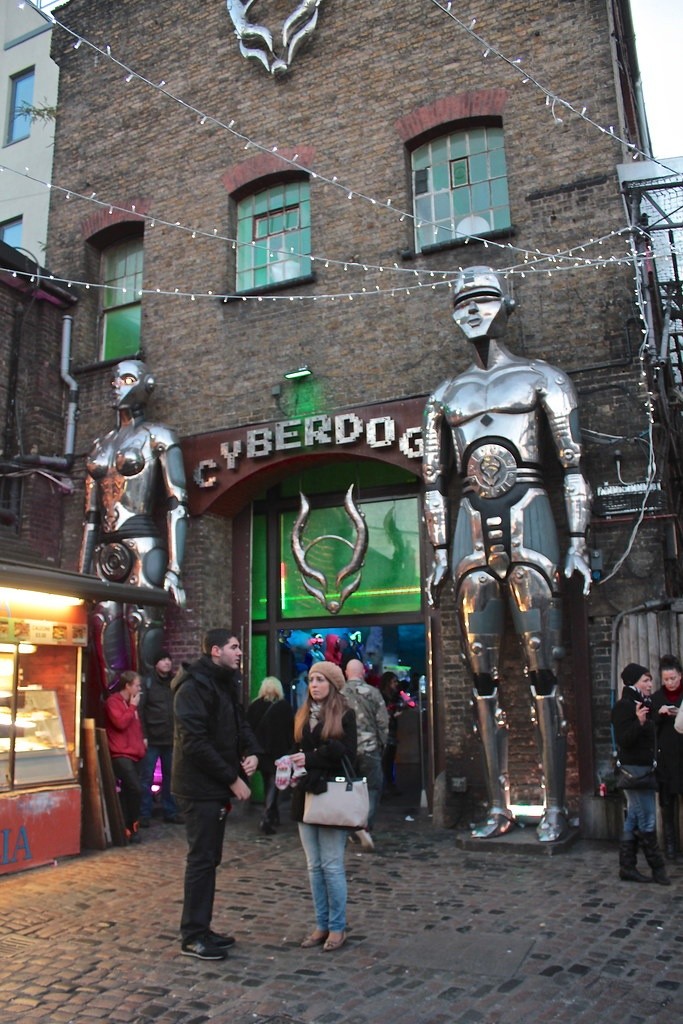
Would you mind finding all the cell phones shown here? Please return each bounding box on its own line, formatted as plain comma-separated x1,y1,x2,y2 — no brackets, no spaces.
668,706,677,712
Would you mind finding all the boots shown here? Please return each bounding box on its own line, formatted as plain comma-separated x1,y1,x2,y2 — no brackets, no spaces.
639,831,671,885
618,839,652,882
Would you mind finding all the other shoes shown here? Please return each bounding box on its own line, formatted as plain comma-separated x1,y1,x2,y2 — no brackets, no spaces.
355,829,375,850
665,844,676,859
124,821,142,843
261,816,279,834
301,929,329,948
324,930,346,950
139,814,151,828
162,815,185,824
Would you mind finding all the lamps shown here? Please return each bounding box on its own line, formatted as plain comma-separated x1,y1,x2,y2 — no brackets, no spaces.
284,368,311,379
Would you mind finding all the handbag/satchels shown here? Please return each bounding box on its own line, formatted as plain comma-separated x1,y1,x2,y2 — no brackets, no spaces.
302,740,370,828
616,765,657,789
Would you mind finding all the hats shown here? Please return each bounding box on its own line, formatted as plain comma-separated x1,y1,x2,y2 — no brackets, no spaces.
621,663,650,686
308,662,345,691
153,650,171,665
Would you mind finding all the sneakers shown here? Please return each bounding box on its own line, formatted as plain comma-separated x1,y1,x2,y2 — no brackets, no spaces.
203,929,235,950
181,931,227,959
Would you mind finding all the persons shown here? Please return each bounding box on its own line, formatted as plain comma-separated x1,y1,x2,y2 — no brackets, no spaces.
172,628,258,962
377,672,399,776
423,265,591,841
612,663,670,885
247,676,296,836
279,629,308,713
653,653,683,858
79,360,188,695
338,659,389,850
138,652,186,827
306,633,364,668
106,672,147,845
276,661,356,950
